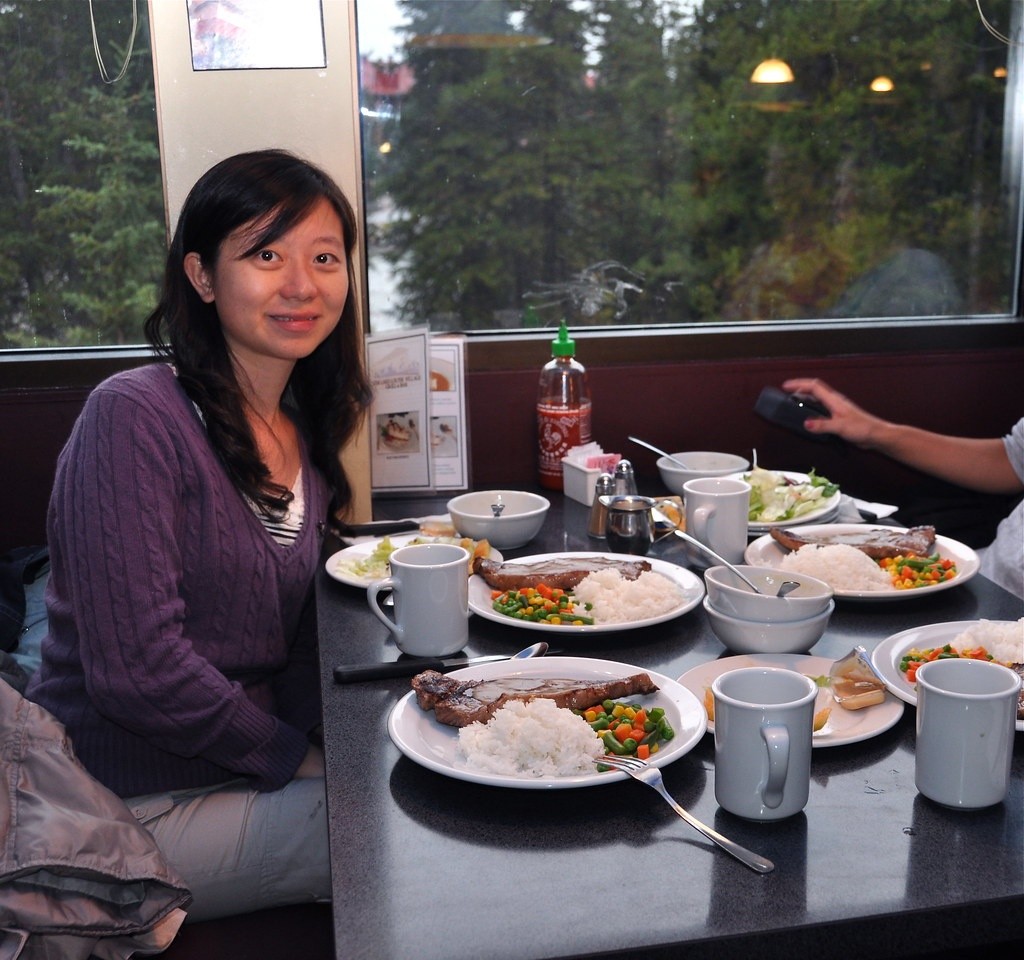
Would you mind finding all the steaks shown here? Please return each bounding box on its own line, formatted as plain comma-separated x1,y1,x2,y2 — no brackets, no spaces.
411,669,661,726
768,524,936,561
470,557,652,588
1006,663,1024,720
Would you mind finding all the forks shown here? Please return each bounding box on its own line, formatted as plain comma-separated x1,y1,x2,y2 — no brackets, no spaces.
592,754,775,873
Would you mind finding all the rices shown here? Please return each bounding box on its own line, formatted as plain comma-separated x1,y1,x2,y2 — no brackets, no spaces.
948,617,1024,666
565,566,679,626
777,542,896,592
457,696,608,779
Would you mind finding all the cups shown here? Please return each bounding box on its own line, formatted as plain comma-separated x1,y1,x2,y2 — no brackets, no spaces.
711,666,819,821
681,477,752,571
915,658,1022,808
366,543,471,658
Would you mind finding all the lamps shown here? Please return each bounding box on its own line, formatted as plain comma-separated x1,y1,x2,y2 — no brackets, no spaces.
405,1,558,49
751,59,795,83
871,75,895,91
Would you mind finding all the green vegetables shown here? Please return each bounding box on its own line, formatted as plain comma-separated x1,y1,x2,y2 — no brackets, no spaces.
335,536,449,579
745,447,840,522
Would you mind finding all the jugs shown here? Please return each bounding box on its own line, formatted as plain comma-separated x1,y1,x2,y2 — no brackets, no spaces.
598,494,683,556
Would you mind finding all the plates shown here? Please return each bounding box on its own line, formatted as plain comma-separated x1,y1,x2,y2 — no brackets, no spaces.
723,470,841,536
871,619,1024,731
325,536,504,591
743,523,982,604
431,357,455,391
467,550,705,633
387,656,709,789
676,653,905,748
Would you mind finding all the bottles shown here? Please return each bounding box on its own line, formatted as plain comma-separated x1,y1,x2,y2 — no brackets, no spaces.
536,319,593,490
586,473,616,539
612,459,639,496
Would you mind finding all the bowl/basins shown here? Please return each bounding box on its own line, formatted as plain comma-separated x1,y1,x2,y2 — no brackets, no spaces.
702,566,836,654
446,490,551,550
655,451,750,498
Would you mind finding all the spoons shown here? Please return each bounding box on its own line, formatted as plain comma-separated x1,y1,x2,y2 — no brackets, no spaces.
509,642,548,661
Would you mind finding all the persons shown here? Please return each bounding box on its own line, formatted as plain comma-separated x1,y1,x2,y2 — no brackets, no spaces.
21,148,373,923
715,135,965,318
781,377,1024,596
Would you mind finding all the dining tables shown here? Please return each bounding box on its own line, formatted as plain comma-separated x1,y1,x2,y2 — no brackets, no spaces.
312,481,1024,960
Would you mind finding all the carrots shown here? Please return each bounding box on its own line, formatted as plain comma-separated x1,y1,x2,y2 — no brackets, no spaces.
906,646,985,681
491,583,574,613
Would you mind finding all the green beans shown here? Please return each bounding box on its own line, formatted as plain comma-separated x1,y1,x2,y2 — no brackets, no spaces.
573,699,674,773
492,590,595,625
900,644,995,671
875,552,957,589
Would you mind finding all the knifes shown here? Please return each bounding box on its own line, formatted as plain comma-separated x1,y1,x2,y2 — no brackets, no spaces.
333,650,562,683
